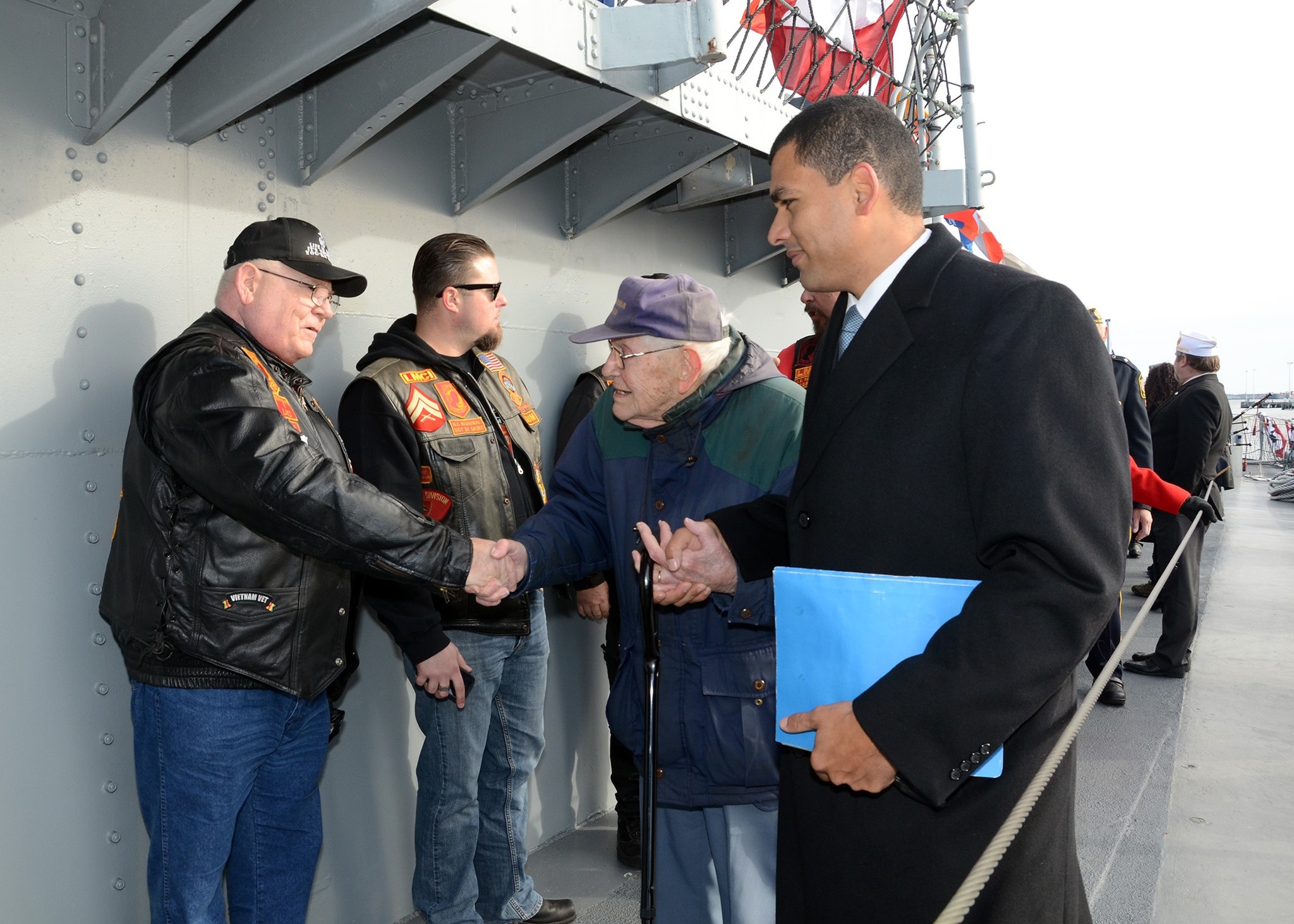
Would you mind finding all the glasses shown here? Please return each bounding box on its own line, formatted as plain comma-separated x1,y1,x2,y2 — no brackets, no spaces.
607,339,687,370
436,282,501,301
256,268,342,314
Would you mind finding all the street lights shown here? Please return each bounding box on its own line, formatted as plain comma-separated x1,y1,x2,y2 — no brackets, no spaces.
1245,370,1248,401
1287,362,1293,402
1253,369,1255,401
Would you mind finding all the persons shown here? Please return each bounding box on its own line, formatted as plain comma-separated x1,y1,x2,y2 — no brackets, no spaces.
554,364,643,867
464,274,809,924
337,232,576,924
631,95,1133,924
771,289,840,392
101,217,517,924
1086,310,1234,706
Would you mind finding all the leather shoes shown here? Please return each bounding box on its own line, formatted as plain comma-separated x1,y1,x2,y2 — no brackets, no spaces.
1131,652,1192,672
519,898,577,924
1091,676,1126,704
1122,658,1185,679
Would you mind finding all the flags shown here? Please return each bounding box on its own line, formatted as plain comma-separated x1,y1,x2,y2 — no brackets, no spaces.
1258,415,1287,458
1285,421,1294,451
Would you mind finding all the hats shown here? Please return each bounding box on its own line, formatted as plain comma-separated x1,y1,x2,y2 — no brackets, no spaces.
1087,308,1102,324
224,217,368,297
568,273,731,344
1175,331,1218,357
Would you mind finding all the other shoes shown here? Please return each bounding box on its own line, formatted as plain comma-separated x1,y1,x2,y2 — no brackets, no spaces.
1128,543,1142,557
1131,580,1154,597
1152,600,1164,614
616,811,642,870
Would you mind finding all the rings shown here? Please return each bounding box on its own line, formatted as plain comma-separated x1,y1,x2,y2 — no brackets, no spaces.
438,685,450,692
658,571,661,584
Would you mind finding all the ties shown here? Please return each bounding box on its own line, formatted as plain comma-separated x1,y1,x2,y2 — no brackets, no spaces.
838,305,866,363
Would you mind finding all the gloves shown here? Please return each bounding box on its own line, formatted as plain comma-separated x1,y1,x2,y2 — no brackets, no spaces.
1178,496,1217,527
